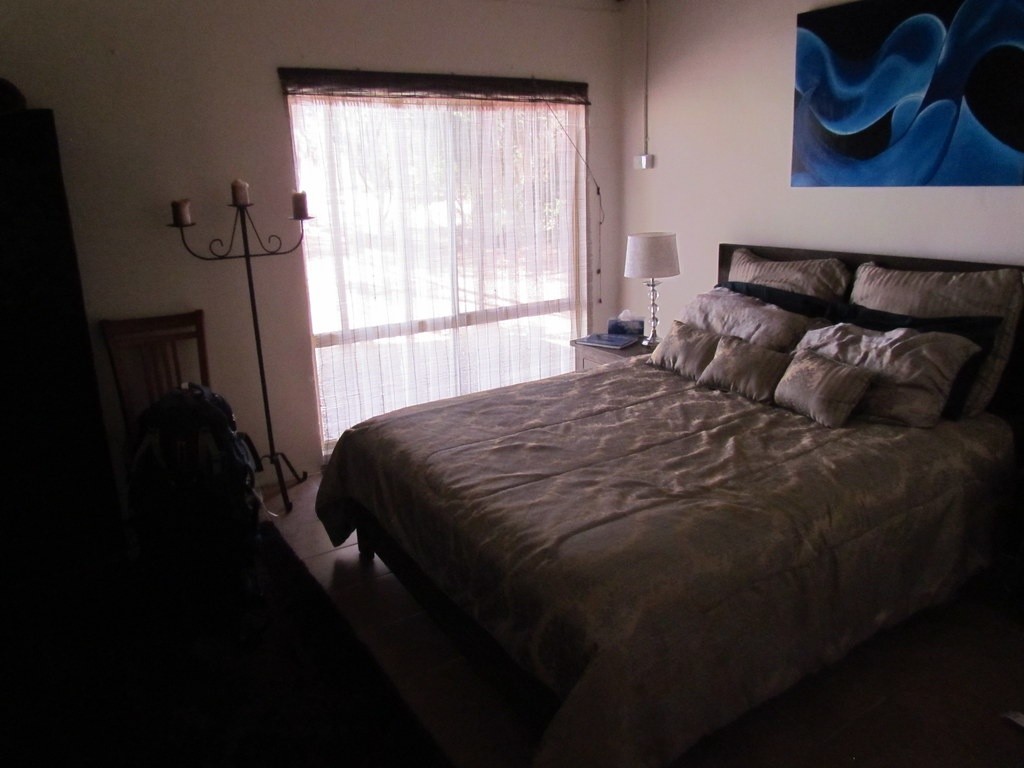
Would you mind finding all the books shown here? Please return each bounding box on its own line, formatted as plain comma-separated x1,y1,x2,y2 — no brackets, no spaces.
575,334,638,349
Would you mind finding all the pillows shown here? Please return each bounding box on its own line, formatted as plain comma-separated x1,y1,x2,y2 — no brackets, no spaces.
646,320,721,380
851,262,1024,418
714,280,830,320
789,319,983,427
829,300,1004,421
679,287,824,353
774,348,879,429
729,248,850,300
696,334,796,408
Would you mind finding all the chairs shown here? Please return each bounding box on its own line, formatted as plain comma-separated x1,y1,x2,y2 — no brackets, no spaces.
101,308,251,534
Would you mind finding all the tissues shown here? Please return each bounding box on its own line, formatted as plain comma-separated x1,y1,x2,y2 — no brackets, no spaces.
608,309,645,335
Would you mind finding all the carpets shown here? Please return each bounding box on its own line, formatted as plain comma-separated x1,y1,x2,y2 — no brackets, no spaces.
132,518,452,768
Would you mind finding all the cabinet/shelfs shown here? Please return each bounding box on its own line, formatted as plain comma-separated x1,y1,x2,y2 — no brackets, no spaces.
0,78,130,589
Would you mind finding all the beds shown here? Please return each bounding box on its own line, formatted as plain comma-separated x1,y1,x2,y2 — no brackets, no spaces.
316,245,1024,768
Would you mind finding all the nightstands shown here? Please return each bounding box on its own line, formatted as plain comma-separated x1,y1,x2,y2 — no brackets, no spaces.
570,338,658,370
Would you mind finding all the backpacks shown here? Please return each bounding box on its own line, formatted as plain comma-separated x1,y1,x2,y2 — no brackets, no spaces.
126,384,260,568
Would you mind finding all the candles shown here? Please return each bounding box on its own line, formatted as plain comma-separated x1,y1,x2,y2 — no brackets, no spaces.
291,193,306,217
230,179,250,206
170,199,194,226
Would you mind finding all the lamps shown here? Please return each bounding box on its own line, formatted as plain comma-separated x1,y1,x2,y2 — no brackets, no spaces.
623,231,681,346
